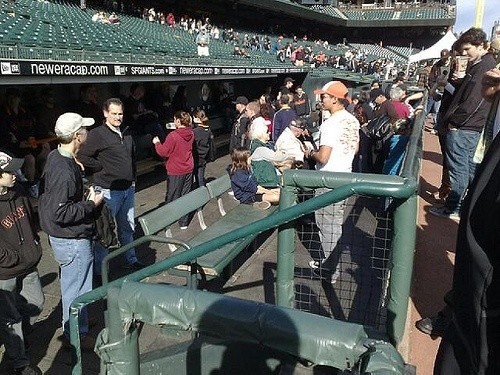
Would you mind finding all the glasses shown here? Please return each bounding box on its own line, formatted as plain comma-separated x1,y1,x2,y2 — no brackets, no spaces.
291,123,299,128
265,129,269,134
301,126,305,131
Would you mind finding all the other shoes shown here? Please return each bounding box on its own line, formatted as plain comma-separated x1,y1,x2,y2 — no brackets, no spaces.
426,189,448,203
425,127,438,134
11,268,130,375
416,315,447,336
180,226,187,229
253,201,271,210
308,261,341,284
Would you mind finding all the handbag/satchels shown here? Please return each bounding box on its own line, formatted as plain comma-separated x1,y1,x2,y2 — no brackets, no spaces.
365,113,391,141
84,189,118,249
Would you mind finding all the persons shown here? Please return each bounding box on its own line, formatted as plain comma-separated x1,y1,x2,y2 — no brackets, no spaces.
0,0,500,375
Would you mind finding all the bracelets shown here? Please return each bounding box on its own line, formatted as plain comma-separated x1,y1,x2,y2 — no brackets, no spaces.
308,149,315,159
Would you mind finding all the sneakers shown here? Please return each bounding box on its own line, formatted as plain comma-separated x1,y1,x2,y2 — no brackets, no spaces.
429,204,456,219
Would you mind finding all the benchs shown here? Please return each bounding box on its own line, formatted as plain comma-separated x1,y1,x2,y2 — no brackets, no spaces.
0,0,458,288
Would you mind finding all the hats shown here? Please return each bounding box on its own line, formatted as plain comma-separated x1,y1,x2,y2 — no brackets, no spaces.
440,49,449,58
284,77,295,83
370,88,381,103
55,113,95,136
490,38,500,50
231,96,248,105
0,152,24,172
314,81,348,99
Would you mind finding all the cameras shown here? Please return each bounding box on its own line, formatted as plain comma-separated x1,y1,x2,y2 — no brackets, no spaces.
94,186,102,195
166,123,177,130
